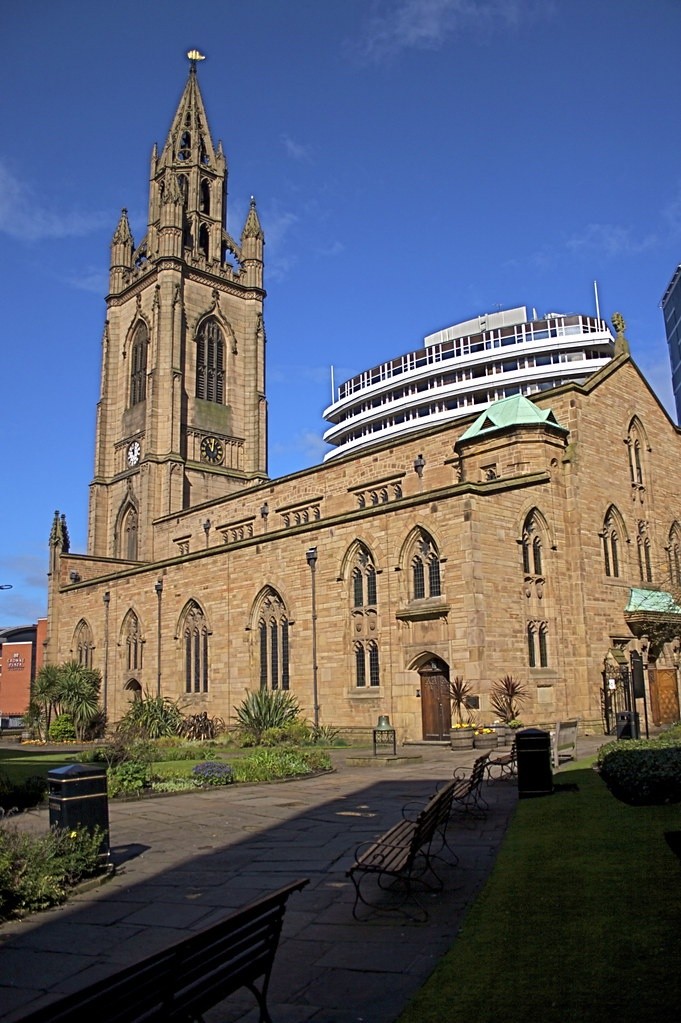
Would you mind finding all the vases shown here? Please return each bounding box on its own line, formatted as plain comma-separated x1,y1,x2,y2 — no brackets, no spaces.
450,721,527,752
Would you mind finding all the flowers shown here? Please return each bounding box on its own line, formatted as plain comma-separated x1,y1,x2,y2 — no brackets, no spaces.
475,728,496,735
451,723,476,729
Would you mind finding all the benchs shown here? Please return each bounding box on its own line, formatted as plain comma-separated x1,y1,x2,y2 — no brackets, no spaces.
429,749,494,831
0,876,312,1023
344,775,460,923
550,720,578,768
486,739,517,787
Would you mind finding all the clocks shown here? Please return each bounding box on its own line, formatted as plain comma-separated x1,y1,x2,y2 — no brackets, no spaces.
126,439,143,468
199,436,226,466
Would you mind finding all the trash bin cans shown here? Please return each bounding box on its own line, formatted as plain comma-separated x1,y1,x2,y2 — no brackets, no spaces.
47,763,111,861
514,728,555,798
616,711,640,741
